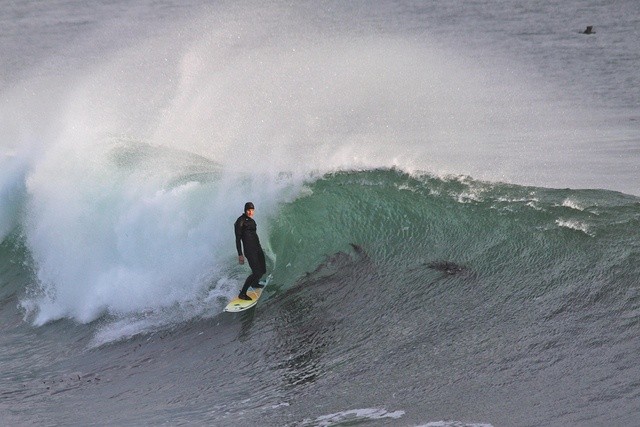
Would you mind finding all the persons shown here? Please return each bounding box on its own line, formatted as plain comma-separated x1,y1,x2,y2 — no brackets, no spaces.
233,201,267,300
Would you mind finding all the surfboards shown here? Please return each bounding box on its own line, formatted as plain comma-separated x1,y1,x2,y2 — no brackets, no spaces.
223,274,272,312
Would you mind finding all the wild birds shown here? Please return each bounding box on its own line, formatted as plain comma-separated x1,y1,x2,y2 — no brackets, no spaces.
577,25,596,34
